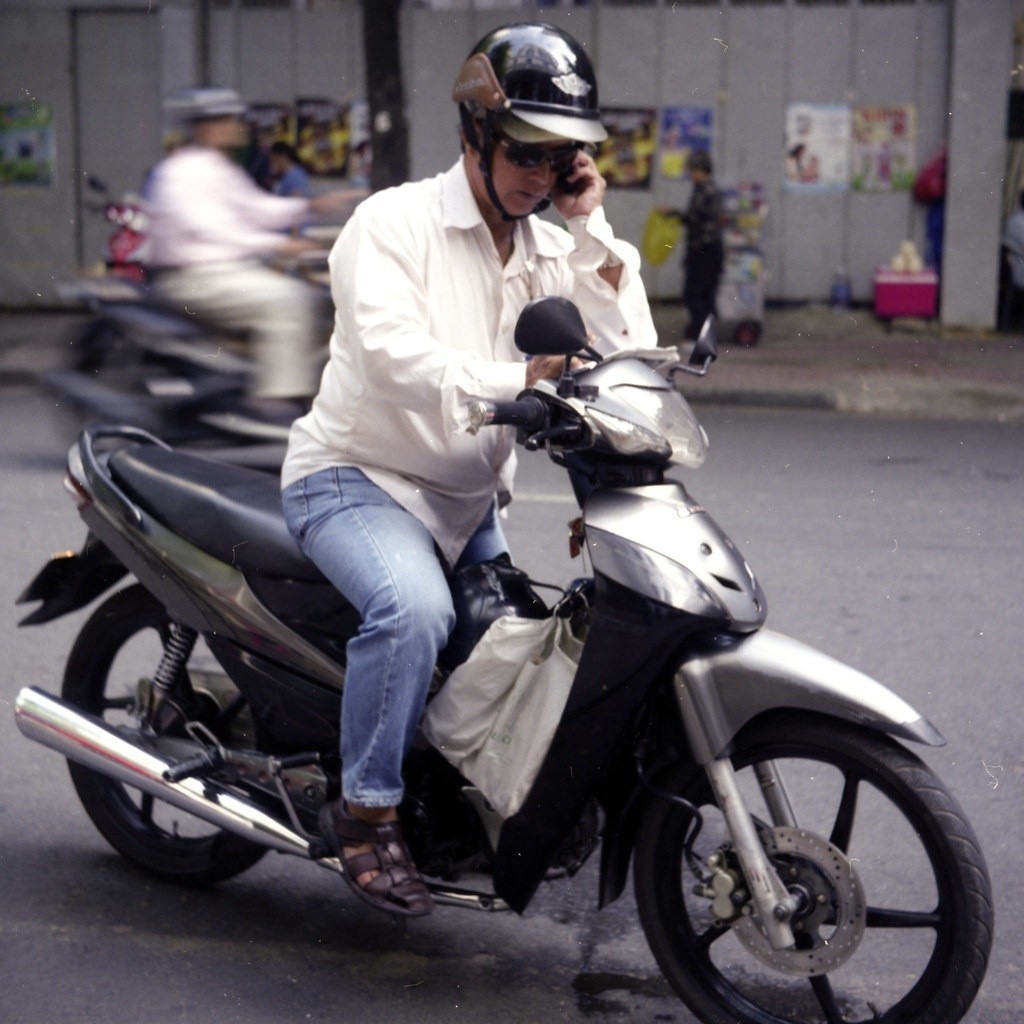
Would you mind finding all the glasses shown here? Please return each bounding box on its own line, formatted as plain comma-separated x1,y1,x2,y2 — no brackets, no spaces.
488,126,579,173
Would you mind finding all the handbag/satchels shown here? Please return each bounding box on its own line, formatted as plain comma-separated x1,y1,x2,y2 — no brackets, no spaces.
421,610,585,821
641,209,682,266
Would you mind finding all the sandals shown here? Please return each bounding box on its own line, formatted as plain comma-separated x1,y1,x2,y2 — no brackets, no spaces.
315,793,438,917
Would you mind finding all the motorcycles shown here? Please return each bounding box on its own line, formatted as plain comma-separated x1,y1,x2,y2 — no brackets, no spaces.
14,292,996,1024
2,249,328,443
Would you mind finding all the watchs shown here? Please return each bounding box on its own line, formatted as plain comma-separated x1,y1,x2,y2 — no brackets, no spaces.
598,251,623,270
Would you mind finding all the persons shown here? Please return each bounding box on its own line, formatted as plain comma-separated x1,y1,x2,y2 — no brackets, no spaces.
280,19,661,918
913,150,943,272
347,139,373,194
265,141,311,278
657,150,726,344
1003,188,1024,332
144,85,372,425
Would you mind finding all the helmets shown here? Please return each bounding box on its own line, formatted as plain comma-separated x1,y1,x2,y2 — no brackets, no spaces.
451,20,611,145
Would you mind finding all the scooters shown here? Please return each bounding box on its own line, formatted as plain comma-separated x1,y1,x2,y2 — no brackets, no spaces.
78,174,155,279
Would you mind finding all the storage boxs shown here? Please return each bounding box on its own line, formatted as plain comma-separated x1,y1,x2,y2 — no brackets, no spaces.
872,272,939,321
